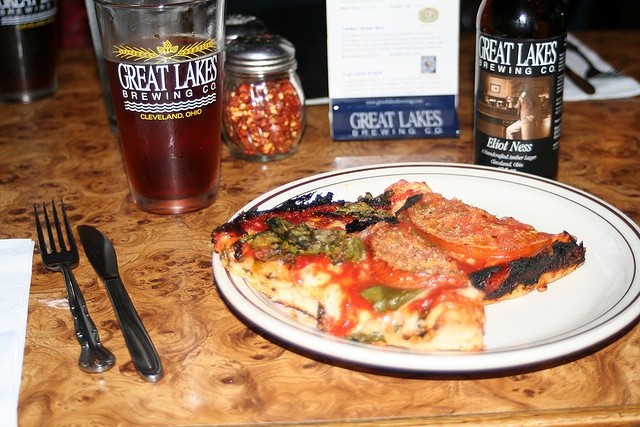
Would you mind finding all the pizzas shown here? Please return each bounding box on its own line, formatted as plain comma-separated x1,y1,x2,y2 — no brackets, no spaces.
210,179,585,351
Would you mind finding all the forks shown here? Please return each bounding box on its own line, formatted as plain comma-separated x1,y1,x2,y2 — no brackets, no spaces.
568,42,627,81
32,198,116,372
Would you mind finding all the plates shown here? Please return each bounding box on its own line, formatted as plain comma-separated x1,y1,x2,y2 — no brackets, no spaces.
212,163,636,375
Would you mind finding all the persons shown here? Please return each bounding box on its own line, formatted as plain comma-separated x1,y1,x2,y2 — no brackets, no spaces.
505,91,537,140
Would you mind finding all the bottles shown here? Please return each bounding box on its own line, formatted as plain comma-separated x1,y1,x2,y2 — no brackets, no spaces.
472,0,567,180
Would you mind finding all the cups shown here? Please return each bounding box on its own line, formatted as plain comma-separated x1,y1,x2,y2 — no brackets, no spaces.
86,2,116,130
93,1,225,215
0,0,63,103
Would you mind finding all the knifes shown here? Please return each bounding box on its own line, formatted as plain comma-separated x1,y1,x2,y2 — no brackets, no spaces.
565,66,596,96
76,226,165,382
